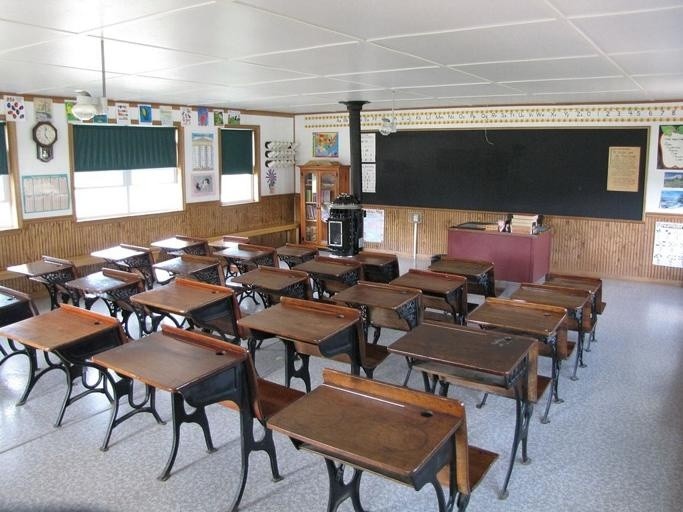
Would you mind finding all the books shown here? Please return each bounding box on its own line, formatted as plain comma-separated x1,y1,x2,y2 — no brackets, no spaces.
509,212,539,235
304,173,336,242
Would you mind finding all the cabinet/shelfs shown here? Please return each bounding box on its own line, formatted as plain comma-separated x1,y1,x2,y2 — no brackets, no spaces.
296,158,352,249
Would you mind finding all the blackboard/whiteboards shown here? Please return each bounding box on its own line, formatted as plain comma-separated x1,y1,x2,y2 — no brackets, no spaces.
358,124,650,223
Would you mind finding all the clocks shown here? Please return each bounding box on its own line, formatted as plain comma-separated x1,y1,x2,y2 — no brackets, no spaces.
32,118,60,162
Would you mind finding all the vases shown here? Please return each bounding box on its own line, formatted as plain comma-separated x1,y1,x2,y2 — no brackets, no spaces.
270,186,277,194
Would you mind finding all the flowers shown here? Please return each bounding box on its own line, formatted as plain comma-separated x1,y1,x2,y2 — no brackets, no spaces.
265,169,277,184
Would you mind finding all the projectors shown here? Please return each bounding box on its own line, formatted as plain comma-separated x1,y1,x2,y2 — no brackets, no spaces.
379,124,397,136
